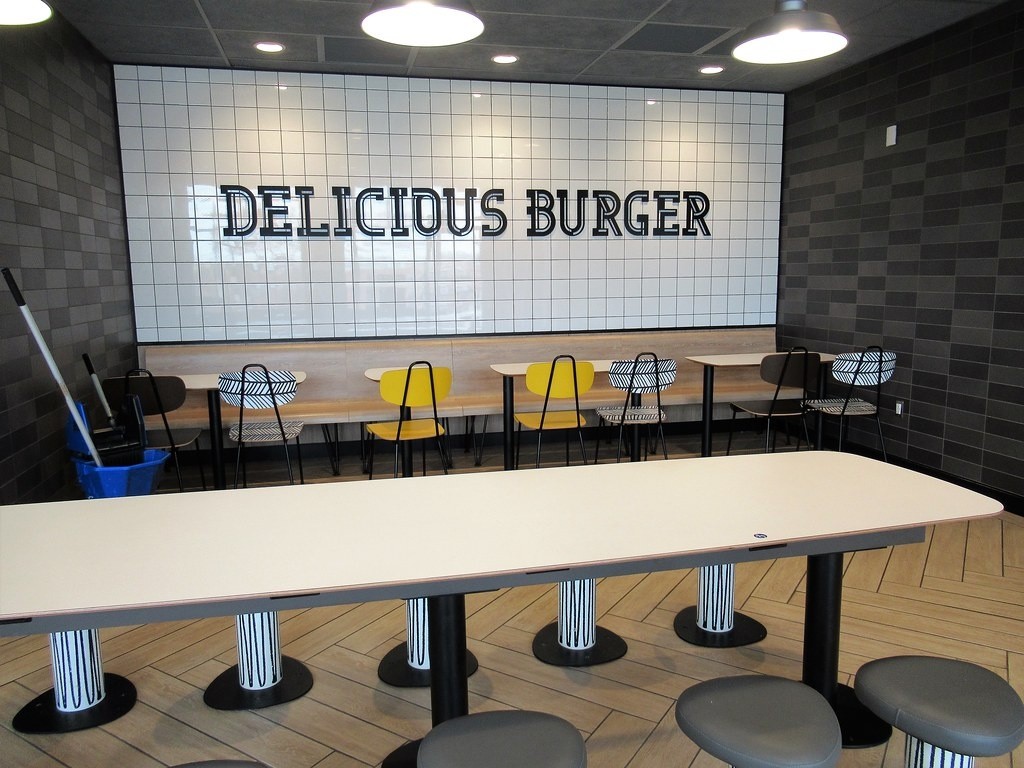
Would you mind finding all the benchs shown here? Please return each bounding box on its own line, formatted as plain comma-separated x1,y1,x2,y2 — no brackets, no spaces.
142,342,350,477
345,341,464,476
452,328,802,468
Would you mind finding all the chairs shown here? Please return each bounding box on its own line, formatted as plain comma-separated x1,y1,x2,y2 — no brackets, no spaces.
102,369,204,491
366,362,449,479
596,352,677,463
218,364,305,490
801,345,898,463
514,354,595,468
725,347,823,455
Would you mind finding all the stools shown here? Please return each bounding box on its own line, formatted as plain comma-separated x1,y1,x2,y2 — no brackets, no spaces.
675,674,842,768
854,656,1024,768
417,709,587,768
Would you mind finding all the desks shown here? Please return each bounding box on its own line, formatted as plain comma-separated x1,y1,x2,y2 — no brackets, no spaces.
685,351,840,460
491,360,645,472
364,367,428,478
117,371,307,491
0,450,1004,768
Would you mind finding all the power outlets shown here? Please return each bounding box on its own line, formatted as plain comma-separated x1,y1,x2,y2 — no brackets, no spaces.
895,401,904,419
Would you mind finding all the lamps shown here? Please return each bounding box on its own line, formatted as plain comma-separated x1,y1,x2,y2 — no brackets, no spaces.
0,0,55,29
358,0,486,47
729,0,850,64
